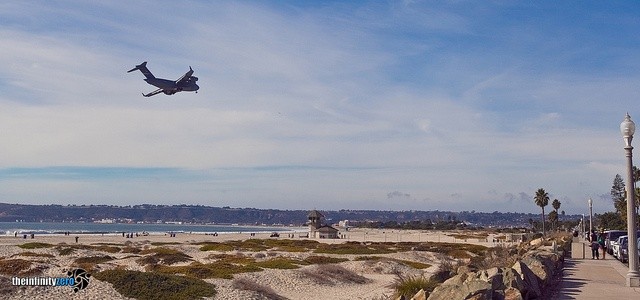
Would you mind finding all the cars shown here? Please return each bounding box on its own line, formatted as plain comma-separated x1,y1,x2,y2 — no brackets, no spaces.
613,235,628,263
594,231,609,251
604,229,627,255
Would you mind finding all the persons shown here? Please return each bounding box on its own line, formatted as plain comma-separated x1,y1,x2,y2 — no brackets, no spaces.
598,228,607,260
590,229,599,260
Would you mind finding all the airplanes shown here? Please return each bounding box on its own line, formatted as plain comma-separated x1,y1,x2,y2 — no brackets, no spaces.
126,59,201,98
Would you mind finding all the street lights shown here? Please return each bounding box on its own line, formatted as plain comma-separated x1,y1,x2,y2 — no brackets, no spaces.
619,113,640,287
588,197,593,242
